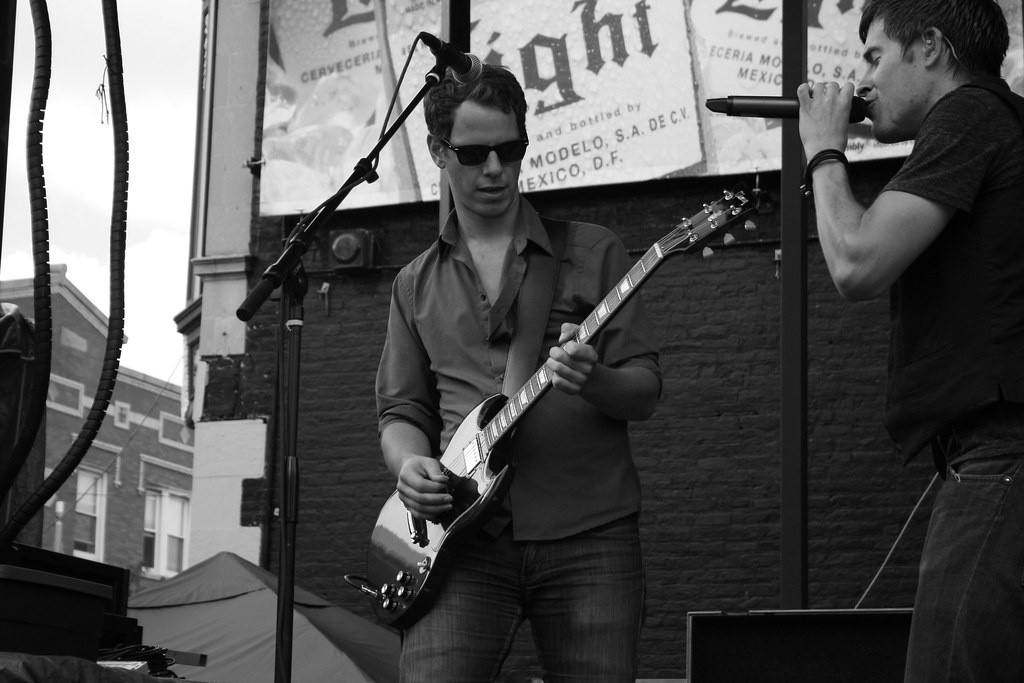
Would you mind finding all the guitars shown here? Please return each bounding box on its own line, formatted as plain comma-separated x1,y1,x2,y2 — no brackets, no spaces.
365,186,779,632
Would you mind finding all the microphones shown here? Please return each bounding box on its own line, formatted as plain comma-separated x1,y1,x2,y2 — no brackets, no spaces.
419,32,481,84
707,97,867,125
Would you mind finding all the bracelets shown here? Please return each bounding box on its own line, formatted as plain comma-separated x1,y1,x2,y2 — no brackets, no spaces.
802,148,849,193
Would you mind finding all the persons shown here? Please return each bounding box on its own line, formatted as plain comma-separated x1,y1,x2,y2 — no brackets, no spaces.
376,63,662,683
797,1,1023,682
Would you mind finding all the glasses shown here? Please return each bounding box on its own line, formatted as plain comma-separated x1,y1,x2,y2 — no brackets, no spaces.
441,126,530,166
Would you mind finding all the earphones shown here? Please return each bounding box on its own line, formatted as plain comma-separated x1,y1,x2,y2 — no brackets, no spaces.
924,40,936,57
